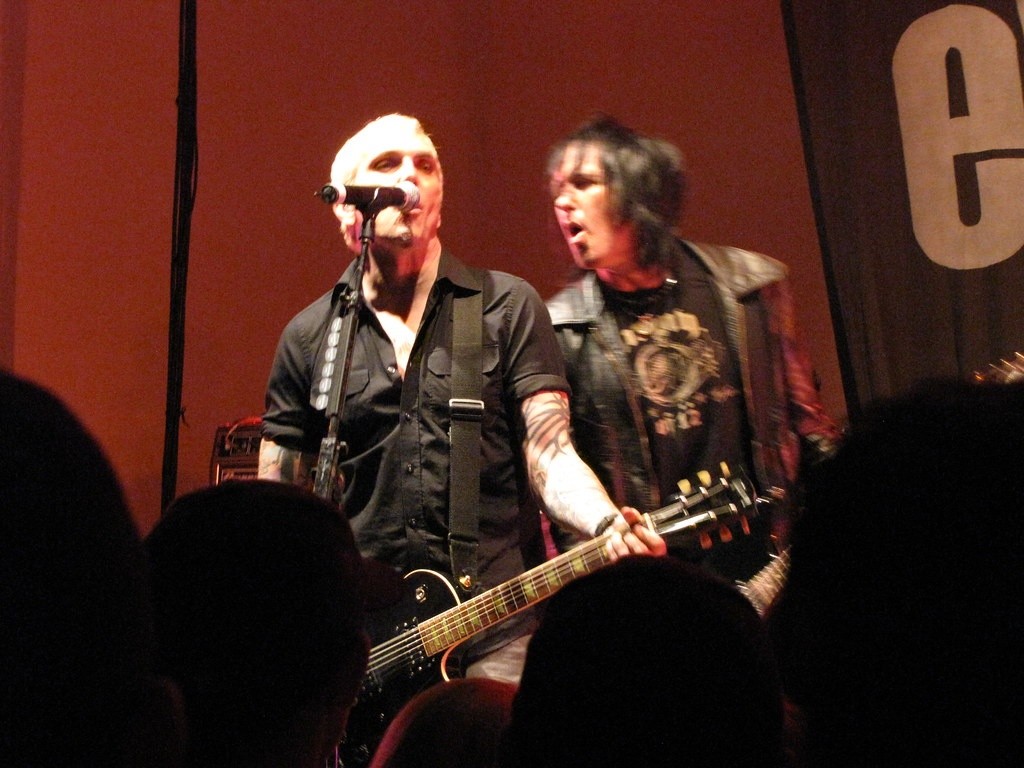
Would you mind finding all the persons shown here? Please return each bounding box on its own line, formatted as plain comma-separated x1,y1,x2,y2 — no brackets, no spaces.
256,112,668,768
0,359,1024,768
543,112,843,768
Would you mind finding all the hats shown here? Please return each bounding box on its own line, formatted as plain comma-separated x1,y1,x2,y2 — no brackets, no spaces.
372,676,521,768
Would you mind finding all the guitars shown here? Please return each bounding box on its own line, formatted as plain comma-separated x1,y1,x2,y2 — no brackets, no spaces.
340,460,762,767
732,349,1023,619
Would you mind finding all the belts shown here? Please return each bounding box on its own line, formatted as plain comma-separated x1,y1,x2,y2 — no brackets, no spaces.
698,514,750,549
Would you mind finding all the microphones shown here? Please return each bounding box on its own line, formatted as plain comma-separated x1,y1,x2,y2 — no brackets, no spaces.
311,181,422,213
